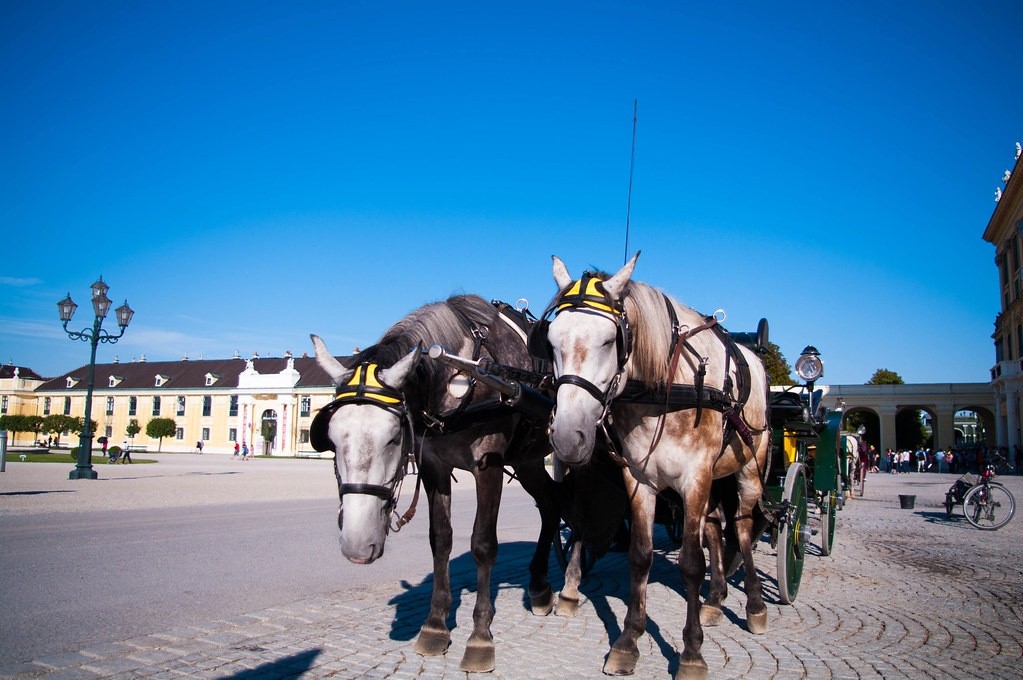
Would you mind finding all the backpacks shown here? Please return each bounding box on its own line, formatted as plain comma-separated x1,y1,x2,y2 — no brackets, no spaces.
918,451,925,461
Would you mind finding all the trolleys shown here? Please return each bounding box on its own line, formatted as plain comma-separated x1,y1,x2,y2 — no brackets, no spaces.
107,445,131,463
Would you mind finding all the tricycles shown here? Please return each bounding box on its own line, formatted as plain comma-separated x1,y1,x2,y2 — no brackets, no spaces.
943,445,1016,531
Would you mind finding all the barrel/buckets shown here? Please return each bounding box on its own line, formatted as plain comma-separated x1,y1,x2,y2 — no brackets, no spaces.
898,495,916,509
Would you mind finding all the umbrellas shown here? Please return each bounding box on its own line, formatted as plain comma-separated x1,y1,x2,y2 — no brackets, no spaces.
97,437,107,443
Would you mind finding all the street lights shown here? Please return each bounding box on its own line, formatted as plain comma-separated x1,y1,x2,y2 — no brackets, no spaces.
57,274,135,479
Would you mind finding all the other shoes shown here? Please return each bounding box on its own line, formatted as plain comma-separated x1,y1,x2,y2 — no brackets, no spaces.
923,469,925,471
918,469,920,472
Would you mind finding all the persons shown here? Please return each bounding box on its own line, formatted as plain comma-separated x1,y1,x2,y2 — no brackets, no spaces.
121,441,133,464
241,442,249,461
250,445,254,458
915,445,961,474
867,445,880,474
196,441,204,454
43,435,57,448
885,449,910,474
102,439,108,457
230,440,241,460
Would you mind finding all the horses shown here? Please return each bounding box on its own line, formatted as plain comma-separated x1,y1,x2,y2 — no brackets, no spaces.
544,253,769,680
845,436,859,502
309,293,615,674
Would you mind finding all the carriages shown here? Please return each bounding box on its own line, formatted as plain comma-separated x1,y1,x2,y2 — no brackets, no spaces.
309,248,869,680
